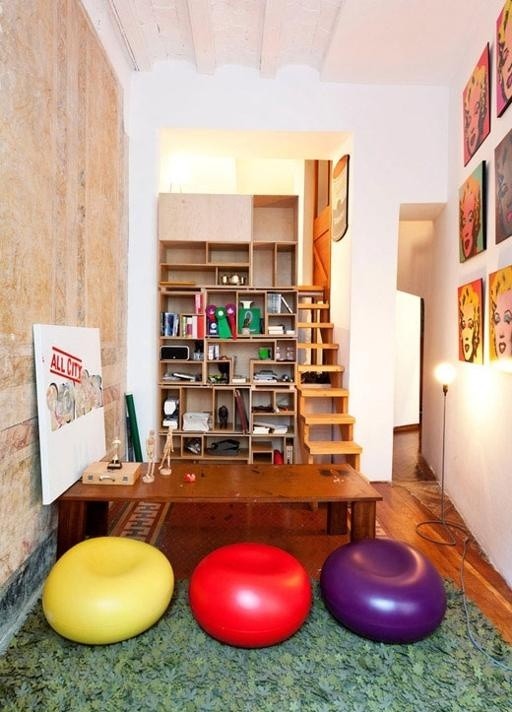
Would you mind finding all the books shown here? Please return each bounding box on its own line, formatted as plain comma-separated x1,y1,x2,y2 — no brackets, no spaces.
231,370,280,383
269,326,295,334
268,294,293,313
162,371,196,382
253,422,287,434
160,293,204,339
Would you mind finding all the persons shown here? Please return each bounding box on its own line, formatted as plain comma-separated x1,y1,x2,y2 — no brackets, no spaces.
489,268,512,362
459,285,480,361
464,65,487,155
496,135,512,235
460,176,480,258
497,0,512,103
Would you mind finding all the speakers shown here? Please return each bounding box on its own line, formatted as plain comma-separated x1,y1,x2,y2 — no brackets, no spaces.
160,345,190,361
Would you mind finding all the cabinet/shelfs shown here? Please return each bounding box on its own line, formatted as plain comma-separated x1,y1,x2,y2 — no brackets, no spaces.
157,191,300,467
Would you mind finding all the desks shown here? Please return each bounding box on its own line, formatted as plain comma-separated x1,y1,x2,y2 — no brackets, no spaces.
54,463,384,566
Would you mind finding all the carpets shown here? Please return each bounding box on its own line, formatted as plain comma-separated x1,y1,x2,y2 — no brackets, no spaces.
0,577,512,711
